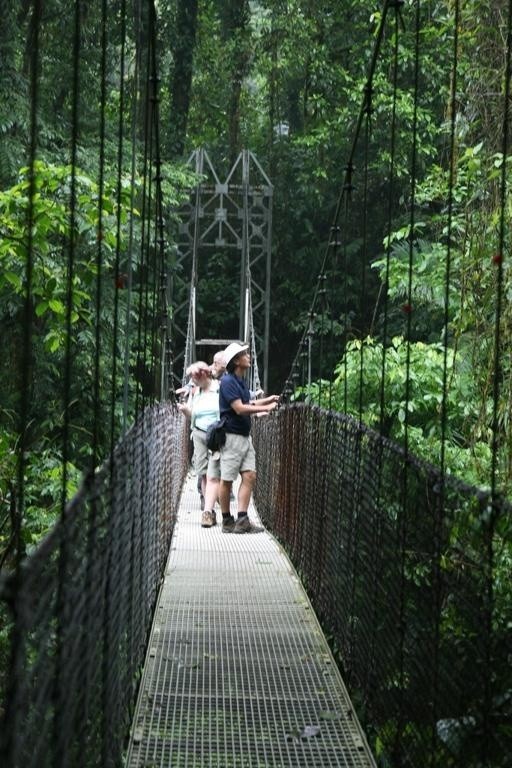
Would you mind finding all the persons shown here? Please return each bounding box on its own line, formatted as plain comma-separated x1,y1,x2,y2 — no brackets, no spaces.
175,352,236,505
217,341,280,534
176,360,269,528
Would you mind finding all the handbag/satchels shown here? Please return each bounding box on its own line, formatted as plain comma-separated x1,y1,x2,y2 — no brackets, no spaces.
206,421,227,452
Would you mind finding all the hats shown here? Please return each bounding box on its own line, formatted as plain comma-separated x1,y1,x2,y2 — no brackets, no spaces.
221,343,250,368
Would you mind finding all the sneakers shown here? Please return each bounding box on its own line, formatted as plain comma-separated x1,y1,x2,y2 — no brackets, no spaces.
198,487,205,511
230,490,235,501
222,515,264,534
201,510,216,527
